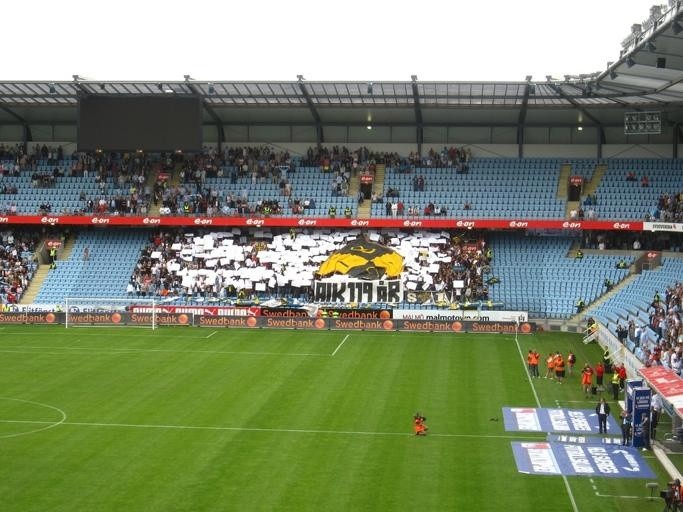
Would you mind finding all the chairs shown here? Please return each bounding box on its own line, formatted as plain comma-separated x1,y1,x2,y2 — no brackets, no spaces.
0,154,682,359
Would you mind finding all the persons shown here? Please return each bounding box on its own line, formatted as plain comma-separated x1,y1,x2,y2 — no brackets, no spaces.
0,138,495,310
524,313,683,512
573,172,681,313
413,410,428,437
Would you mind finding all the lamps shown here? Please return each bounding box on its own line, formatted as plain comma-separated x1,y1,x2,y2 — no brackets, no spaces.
593,18,683,91
